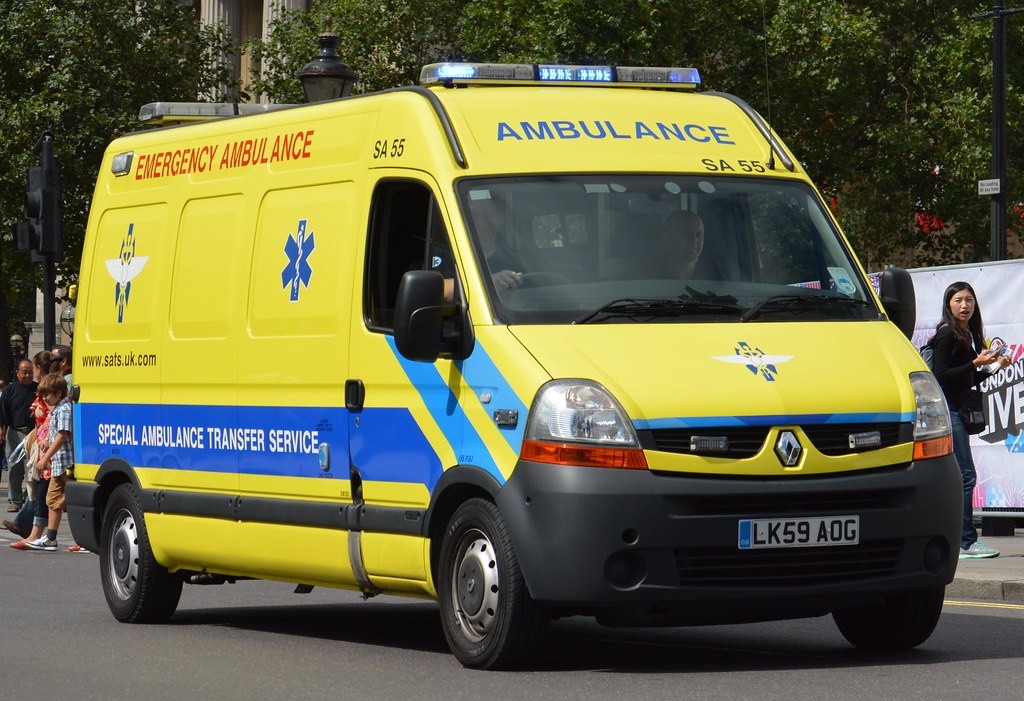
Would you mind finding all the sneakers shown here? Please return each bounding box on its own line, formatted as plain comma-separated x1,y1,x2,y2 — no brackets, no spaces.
79,547,92,554
26,535,58,551
3,520,31,538
958,540,1000,559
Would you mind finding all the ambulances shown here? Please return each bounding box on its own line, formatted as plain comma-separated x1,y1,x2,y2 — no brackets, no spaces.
62,60,965,672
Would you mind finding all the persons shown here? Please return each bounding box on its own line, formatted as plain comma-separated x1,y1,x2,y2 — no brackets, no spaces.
624,210,721,280
927,282,1013,558
0,344,92,554
428,192,535,304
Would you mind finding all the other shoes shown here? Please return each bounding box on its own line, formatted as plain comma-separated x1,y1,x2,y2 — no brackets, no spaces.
68,544,81,551
4,468,8,471
10,540,31,549
8,502,19,512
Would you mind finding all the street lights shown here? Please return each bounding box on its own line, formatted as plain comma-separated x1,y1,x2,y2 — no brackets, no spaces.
10,334,24,381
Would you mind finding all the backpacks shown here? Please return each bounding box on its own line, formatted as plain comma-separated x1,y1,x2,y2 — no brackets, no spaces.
920,324,949,372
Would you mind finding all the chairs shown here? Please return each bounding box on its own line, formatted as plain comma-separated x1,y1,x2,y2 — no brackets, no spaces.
386,186,449,329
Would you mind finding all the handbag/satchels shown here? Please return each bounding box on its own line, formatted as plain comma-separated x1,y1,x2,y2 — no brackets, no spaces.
959,371,986,435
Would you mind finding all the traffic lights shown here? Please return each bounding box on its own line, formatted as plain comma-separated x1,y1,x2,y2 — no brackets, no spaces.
12,166,58,262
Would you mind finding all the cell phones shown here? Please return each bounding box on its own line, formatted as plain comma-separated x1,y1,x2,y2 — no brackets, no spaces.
998,343,1006,351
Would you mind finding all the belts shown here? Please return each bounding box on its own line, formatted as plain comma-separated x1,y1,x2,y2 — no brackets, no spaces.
10,427,30,432
